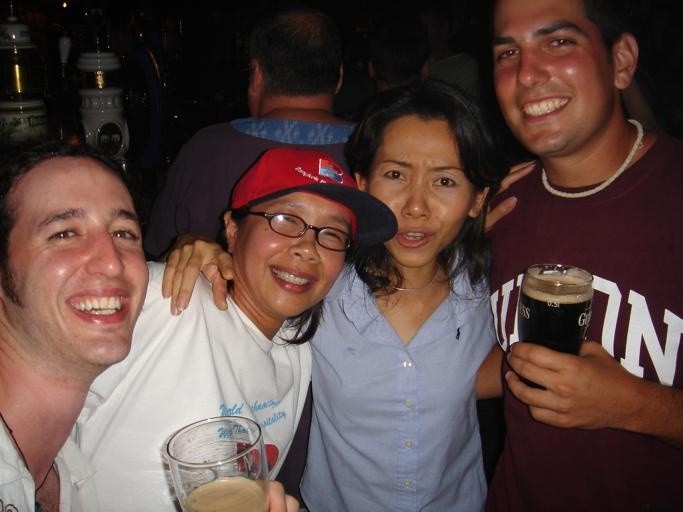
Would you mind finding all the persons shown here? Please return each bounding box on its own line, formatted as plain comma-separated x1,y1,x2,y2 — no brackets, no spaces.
0,139,300,512
142,7,363,265
588,3,668,130
483,2,682,512
361,12,432,94
162,88,535,512
58,148,399,512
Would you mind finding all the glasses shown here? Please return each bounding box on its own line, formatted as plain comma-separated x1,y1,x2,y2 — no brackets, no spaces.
244,209,351,252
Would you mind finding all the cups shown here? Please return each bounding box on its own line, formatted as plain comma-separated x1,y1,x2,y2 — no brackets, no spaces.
167,416,272,512
518,263,594,391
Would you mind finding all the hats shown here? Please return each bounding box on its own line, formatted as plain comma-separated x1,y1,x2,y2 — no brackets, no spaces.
229,147,397,245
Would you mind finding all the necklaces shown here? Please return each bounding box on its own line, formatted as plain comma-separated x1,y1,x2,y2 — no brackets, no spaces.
0,412,53,512
394,265,441,291
